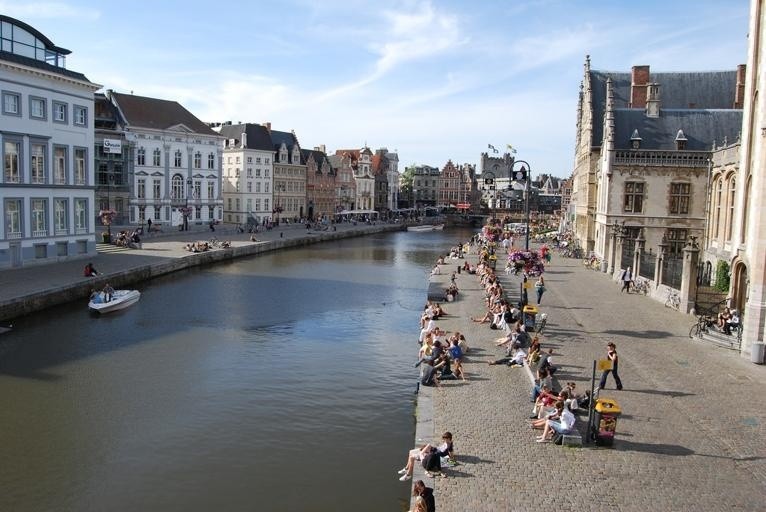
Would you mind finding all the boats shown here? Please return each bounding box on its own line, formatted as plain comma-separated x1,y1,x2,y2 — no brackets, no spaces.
87,287,141,315
407,223,445,232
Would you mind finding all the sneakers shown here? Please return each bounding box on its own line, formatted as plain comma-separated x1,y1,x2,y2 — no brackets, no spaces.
536,436,542,439
398,474,411,482
536,438,547,443
397,468,407,476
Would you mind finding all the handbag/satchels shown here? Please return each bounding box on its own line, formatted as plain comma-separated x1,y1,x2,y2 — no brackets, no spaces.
552,432,562,445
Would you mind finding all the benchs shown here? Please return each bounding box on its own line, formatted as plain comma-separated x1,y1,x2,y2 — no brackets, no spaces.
506,323,583,447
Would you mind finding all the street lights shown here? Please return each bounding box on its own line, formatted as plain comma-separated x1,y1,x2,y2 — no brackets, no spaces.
507,159,532,254
482,172,496,222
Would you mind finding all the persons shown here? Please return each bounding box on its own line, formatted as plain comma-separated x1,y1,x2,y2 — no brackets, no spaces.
469,228,526,264
506,245,551,304
584,254,597,269
102,283,114,303
528,336,557,403
397,430,456,482
620,265,632,293
84,264,97,277
112,230,142,249
720,309,741,336
431,242,476,301
147,218,152,232
412,495,428,512
526,382,594,442
414,479,436,512
185,207,419,254
594,341,623,390
718,306,732,329
413,301,466,389
529,228,584,259
470,260,532,366
88,263,103,276
89,288,102,304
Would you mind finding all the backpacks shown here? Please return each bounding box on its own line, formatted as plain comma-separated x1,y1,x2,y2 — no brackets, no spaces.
421,453,440,471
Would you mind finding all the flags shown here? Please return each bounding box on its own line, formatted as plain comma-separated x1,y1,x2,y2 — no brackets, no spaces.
488,143,518,156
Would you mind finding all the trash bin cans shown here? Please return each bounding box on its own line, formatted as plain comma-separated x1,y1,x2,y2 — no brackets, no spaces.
751,341,766,364
104,235,110,243
601,260,608,272
489,255,497,269
523,305,538,332
179,225,184,231
591,399,621,446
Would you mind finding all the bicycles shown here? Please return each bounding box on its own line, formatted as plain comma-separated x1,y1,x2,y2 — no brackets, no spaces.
689,314,714,339
664,289,680,311
536,313,548,339
629,279,648,296
